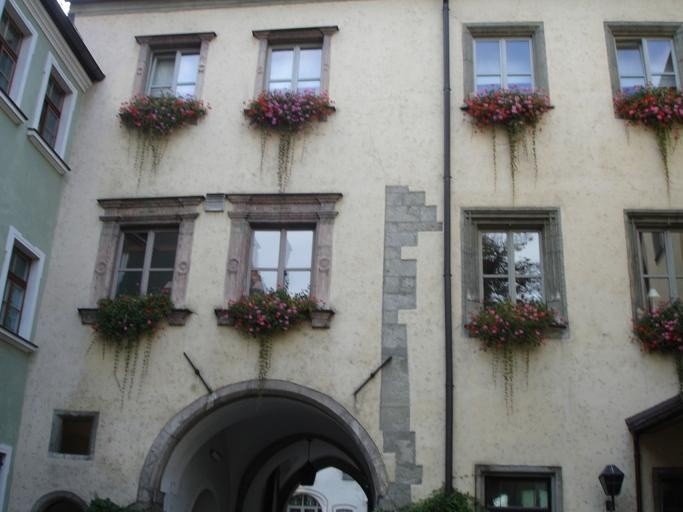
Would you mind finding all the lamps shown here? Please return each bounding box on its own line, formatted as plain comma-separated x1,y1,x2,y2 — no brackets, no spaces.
598,464,624,511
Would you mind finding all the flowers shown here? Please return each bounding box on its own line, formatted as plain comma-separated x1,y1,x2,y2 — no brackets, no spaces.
460,294,681,417
458,86,681,204
80,288,326,407
116,87,335,191
